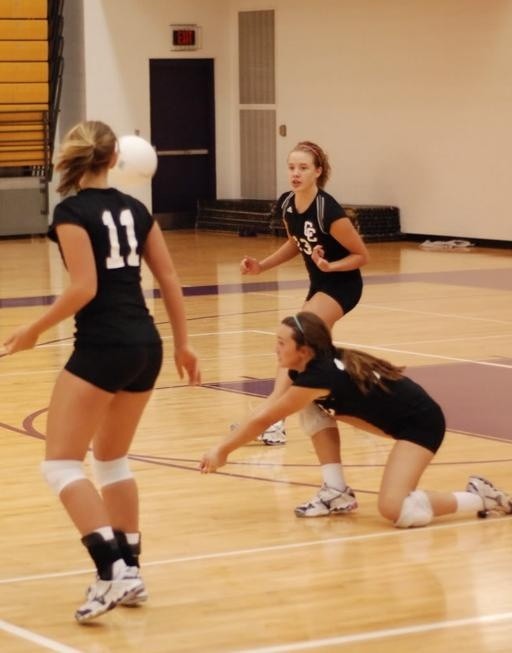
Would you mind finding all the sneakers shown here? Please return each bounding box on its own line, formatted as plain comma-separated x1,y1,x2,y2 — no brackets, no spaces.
294,481,359,518
466,475,511,519
75,559,149,624
229,419,288,445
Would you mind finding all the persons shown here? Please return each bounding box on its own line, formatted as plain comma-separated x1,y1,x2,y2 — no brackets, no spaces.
0,117,201,625
198,311,511,528
231,139,371,446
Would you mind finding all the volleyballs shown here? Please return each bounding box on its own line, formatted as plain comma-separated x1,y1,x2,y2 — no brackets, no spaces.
112,133,158,185
